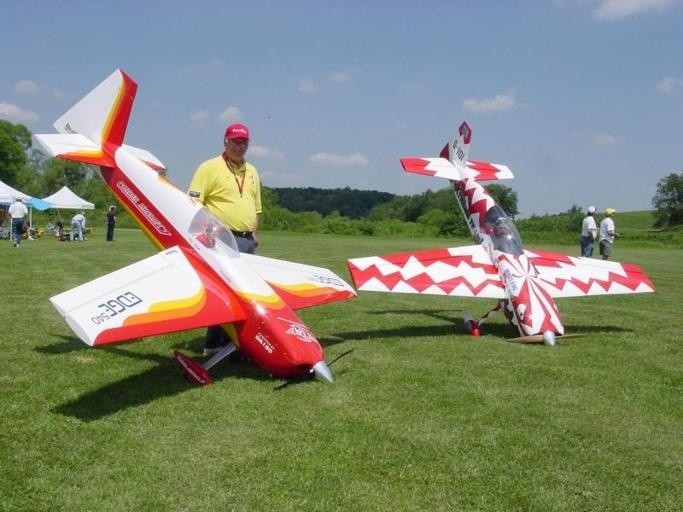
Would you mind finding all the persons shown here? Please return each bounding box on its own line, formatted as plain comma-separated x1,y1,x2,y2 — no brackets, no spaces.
581,206,599,257
599,208,619,260
69,211,86,243
188,122,263,357
105,204,117,242
8,197,33,247
198,221,220,248
493,217,511,252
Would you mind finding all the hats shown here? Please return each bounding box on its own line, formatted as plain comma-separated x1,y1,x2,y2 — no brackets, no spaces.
588,206,596,213
605,207,615,217
224,123,250,140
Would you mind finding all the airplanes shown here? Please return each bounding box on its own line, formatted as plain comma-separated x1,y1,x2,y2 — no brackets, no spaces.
32,66,358,392
345,120,656,347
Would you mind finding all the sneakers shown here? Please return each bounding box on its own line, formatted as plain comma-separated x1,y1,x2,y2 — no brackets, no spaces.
203,347,225,355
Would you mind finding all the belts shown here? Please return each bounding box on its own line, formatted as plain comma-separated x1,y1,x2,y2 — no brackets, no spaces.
231,230,253,239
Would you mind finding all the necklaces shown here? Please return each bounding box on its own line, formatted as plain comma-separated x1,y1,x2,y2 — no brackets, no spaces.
227,158,246,196
